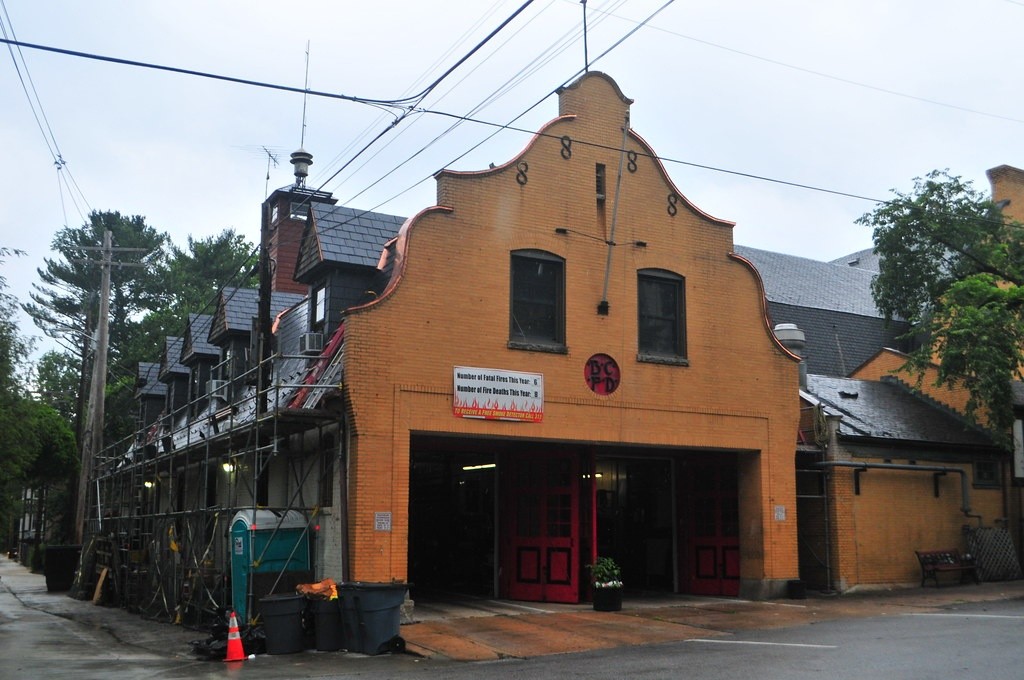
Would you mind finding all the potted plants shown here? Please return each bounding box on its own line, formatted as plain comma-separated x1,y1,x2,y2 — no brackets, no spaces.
584,556,623,611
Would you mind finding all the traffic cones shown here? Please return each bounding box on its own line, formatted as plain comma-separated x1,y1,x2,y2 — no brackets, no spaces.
222,611,248,662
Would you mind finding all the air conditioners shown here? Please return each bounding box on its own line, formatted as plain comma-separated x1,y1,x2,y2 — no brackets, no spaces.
206,380,228,402
299,333,323,353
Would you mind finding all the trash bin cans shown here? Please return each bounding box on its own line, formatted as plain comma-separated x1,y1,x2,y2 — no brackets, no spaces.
301,599,344,652
336,580,414,656
46,545,83,592
258,597,305,655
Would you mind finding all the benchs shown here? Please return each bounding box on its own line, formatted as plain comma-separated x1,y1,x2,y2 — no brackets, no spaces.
914,548,980,586
